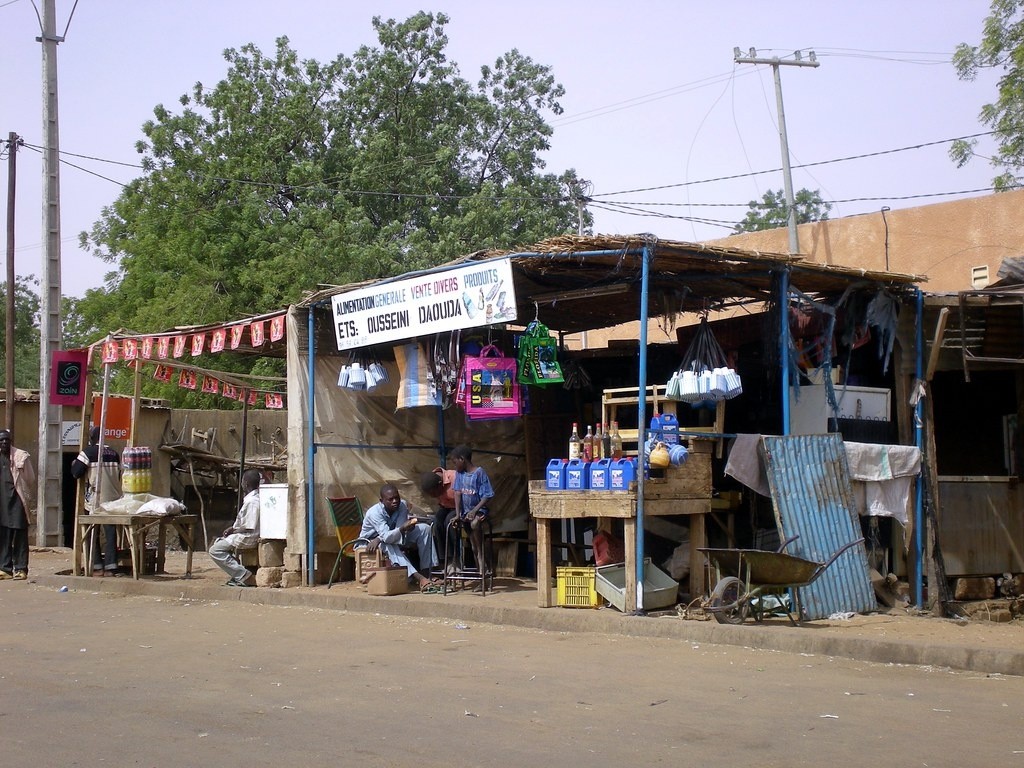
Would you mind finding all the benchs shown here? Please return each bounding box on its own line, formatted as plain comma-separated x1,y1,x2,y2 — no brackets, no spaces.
493,537,592,577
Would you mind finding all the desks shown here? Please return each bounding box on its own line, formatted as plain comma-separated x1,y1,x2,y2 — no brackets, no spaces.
78,515,197,579
528,454,713,608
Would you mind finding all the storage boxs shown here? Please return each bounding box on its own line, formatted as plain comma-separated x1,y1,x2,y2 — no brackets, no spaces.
366,566,409,595
259,483,288,539
592,530,625,566
556,566,603,608
595,560,679,612
118,548,158,575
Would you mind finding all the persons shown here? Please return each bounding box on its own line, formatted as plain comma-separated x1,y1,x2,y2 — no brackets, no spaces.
0,429,36,580
420,467,456,560
208,469,260,586
353,485,449,594
450,445,495,575
71,427,122,577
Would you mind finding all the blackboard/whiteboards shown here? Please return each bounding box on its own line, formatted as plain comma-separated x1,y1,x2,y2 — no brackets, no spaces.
56,361,80,396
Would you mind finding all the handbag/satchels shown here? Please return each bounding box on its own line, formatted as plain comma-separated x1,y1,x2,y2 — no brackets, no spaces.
666,322,742,405
455,345,529,421
559,351,592,392
518,320,564,388
338,350,390,393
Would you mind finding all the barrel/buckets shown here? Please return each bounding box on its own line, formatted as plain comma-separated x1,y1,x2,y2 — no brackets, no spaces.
116,547,133,572
144,548,156,574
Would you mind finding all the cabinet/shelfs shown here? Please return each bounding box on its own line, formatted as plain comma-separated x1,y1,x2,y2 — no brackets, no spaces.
790,386,891,442
600,385,725,458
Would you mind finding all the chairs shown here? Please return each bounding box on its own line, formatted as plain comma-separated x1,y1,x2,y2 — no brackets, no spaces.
324,495,372,590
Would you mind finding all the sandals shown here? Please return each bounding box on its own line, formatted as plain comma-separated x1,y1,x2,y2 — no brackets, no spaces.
429,577,445,586
420,582,437,594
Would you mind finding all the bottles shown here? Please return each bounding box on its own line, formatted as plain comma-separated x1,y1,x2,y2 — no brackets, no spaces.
121,446,152,470
568,421,621,460
651,413,679,443
463,292,478,320
121,469,153,493
486,279,504,302
479,288,484,310
487,304,493,323
337,362,390,392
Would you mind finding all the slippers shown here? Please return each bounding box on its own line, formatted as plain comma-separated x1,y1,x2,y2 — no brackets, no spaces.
226,581,246,586
0,572,12,579
14,572,26,579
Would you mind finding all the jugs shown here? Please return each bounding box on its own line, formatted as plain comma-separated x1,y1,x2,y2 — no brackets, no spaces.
645,433,688,466
546,457,649,490
